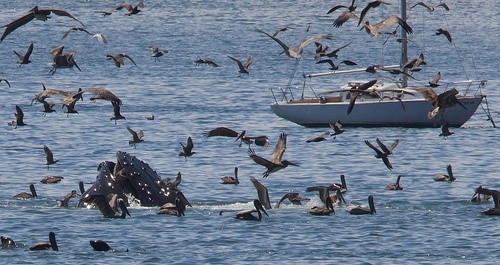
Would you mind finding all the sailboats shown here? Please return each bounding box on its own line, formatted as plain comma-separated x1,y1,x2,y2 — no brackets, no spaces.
269,0,494,128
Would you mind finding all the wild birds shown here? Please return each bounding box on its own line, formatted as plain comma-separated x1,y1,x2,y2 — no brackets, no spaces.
7,104,28,129
227,54,254,78
254,28,333,60
221,167,239,185
0,0,500,251
178,137,197,163
127,126,144,147
106,53,138,68
328,119,345,139
376,137,399,155
246,132,301,178
431,27,451,42
411,3,449,12
147,46,167,62
43,145,59,171
